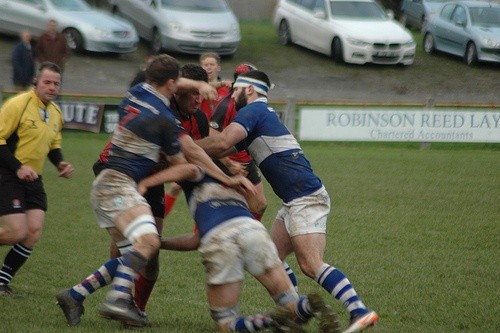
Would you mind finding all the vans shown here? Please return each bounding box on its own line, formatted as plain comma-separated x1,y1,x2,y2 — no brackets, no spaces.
106,0,243,56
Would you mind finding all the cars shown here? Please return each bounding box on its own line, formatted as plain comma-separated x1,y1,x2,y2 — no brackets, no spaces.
272,0,416,66
399,0,500,66
0,0,139,54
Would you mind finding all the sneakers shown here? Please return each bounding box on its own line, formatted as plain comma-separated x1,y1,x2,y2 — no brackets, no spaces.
99,296,151,326
344,310,378,333
306,293,341,333
55,288,85,326
269,306,306,333
0,284,15,296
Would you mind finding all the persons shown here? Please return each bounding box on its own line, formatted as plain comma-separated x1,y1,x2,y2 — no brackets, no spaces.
0,61,75,300
54,52,377,333
36,19,71,90
10,28,37,91
